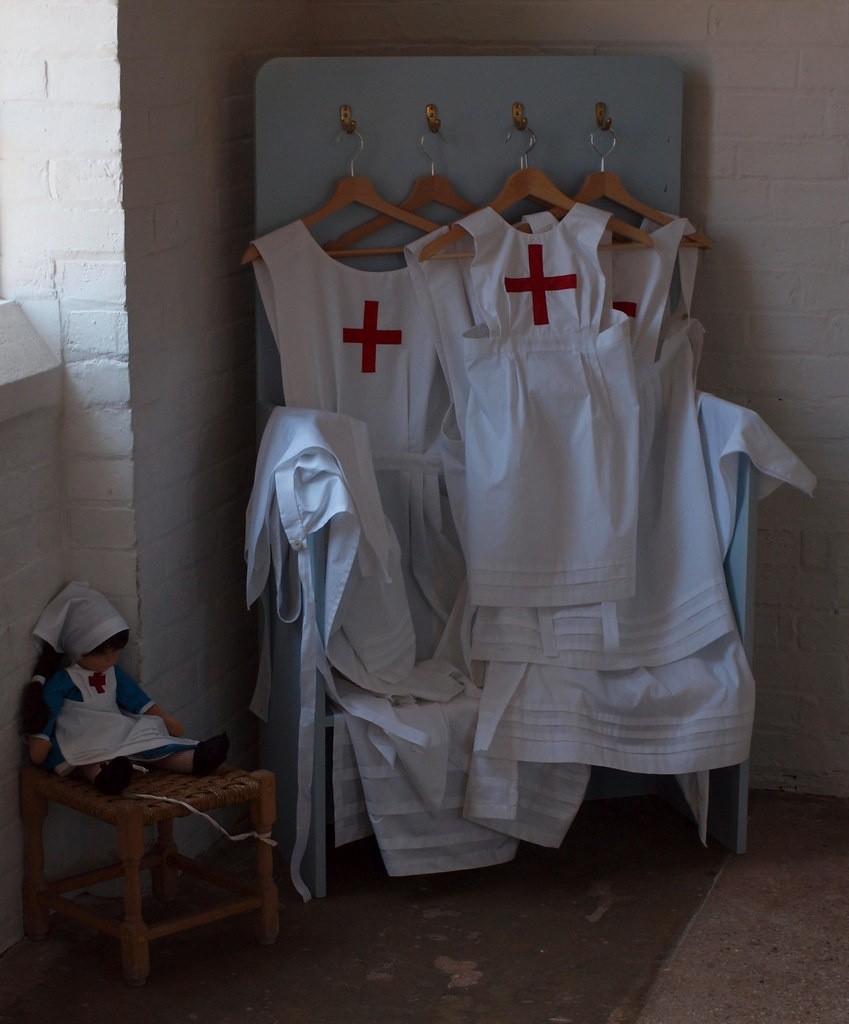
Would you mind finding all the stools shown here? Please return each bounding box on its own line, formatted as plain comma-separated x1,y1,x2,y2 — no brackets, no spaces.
17,755,279,983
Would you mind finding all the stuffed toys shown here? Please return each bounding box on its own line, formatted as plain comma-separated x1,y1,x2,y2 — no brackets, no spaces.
18,580,232,797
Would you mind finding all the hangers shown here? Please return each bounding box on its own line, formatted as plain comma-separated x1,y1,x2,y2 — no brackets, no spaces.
242,117,715,255
